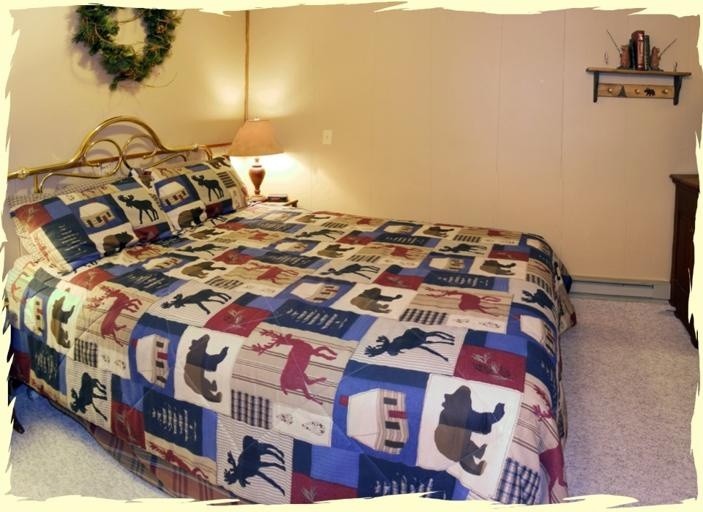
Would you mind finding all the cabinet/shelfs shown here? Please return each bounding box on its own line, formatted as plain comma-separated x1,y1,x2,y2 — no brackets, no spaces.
669,173,698,348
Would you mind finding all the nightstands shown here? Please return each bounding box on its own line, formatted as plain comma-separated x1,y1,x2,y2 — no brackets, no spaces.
247,195,298,207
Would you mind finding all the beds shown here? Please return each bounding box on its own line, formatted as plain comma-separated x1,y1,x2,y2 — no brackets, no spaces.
2,114,576,504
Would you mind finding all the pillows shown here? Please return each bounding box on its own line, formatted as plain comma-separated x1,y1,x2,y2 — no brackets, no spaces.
9,176,177,276
131,156,250,229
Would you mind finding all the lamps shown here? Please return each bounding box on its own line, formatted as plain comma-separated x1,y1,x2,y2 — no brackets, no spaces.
225,119,286,202
